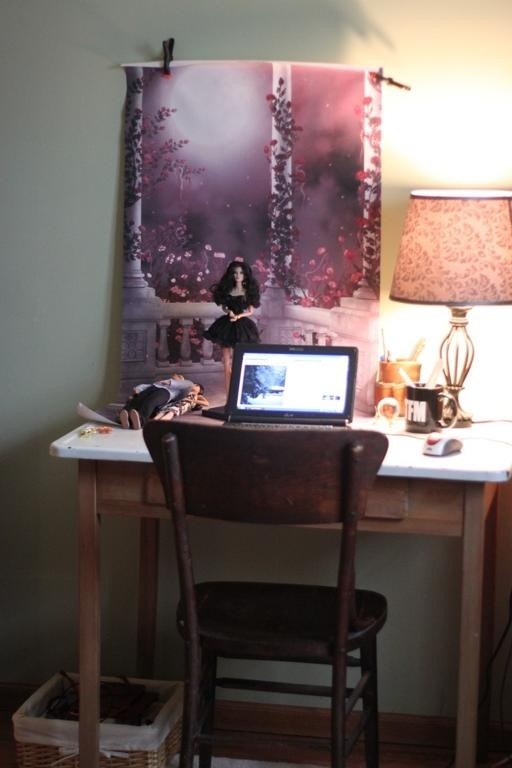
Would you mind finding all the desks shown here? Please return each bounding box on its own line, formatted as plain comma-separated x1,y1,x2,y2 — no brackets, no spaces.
33,379,512,767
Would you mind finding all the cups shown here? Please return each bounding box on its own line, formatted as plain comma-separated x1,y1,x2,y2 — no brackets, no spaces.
381,359,420,417
404,382,457,433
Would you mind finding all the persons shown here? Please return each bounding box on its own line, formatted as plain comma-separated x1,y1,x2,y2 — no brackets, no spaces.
152,382,210,420
206,261,260,404
120,373,193,430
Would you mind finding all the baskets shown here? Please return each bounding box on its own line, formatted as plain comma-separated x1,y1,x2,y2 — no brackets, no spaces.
13,671,185,768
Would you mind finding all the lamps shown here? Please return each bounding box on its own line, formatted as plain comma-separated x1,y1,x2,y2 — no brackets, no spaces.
378,182,512,440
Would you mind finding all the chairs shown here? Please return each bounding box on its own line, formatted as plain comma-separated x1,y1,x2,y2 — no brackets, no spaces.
139,413,397,767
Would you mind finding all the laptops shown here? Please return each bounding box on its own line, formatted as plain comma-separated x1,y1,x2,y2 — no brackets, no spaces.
220,342,358,431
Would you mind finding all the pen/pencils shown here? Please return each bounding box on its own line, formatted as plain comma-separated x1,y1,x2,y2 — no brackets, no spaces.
380,326,427,364
398,359,444,389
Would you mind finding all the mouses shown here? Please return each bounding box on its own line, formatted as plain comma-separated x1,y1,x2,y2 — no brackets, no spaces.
423,435,462,457
